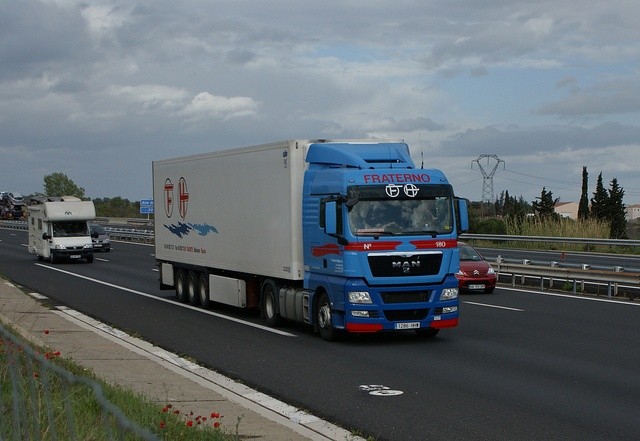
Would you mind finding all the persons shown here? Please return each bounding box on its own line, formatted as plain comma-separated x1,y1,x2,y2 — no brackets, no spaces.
411,201,439,227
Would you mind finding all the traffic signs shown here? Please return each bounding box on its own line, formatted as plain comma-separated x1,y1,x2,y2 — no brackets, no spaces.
140,199,153,206
140,207,153,213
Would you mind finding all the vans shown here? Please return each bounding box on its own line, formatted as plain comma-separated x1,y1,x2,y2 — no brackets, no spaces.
8,191,25,204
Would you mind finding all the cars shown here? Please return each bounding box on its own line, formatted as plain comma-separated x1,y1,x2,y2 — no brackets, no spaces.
455,242,497,295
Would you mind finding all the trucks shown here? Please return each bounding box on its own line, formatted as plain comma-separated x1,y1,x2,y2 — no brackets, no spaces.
27,201,96,264
152,139,469,342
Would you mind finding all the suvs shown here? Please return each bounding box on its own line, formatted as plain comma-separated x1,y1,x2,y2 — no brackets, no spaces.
90,224,110,252
